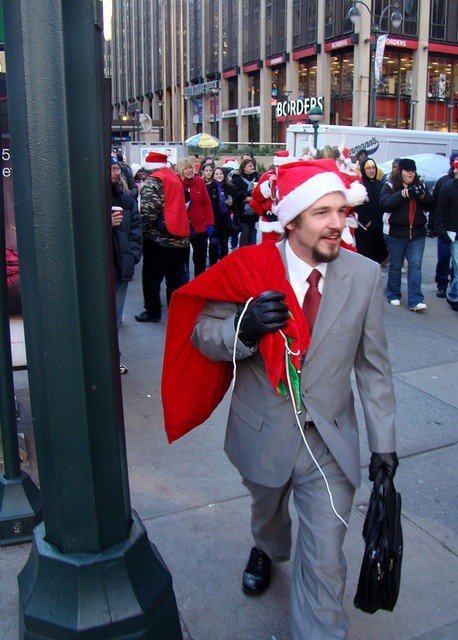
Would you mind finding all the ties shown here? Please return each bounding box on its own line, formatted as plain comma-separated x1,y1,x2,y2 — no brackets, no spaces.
301,269,322,337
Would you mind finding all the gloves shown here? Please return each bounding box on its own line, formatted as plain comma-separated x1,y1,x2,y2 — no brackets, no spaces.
207,226,214,236
402,188,415,198
234,290,289,348
368,453,398,481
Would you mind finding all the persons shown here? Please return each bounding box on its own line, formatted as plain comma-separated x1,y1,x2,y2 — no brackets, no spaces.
200,163,213,190
249,150,292,242
431,152,457,298
354,156,388,264
229,159,261,247
306,133,348,159
433,157,457,312
201,157,213,162
207,166,236,267
381,159,400,182
188,160,399,639
133,151,190,322
118,160,139,198
110,155,143,374
176,158,214,278
379,159,431,309
355,149,383,180
131,163,143,219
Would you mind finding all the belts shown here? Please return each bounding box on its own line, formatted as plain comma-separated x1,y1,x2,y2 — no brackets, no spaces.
304,421,318,430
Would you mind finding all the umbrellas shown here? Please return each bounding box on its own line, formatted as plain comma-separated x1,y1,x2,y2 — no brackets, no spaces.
185,133,224,149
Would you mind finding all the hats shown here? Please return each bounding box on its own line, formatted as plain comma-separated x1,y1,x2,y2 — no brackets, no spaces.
399,159,416,170
141,152,171,170
223,158,238,169
277,159,369,230
273,151,291,165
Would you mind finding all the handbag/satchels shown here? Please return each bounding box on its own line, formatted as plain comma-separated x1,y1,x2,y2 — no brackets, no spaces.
354,468,403,614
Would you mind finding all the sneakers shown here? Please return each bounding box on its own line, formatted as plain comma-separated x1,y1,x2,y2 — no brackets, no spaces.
390,299,400,306
437,289,446,297
409,303,427,311
134,312,161,322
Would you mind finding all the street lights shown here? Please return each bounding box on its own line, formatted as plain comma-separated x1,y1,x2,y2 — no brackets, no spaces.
349,0,403,124
282,90,293,128
210,86,221,138
117,113,127,138
447,103,454,131
155,100,165,141
308,107,324,149
409,100,418,129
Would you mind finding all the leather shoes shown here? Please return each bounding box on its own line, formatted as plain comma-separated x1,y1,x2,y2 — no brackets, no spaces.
242,546,271,596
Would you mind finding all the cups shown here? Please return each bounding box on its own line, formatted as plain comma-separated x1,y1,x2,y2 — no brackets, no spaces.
111,206,123,227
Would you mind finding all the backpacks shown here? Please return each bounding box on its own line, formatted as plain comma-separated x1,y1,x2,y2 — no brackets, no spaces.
241,177,256,216
